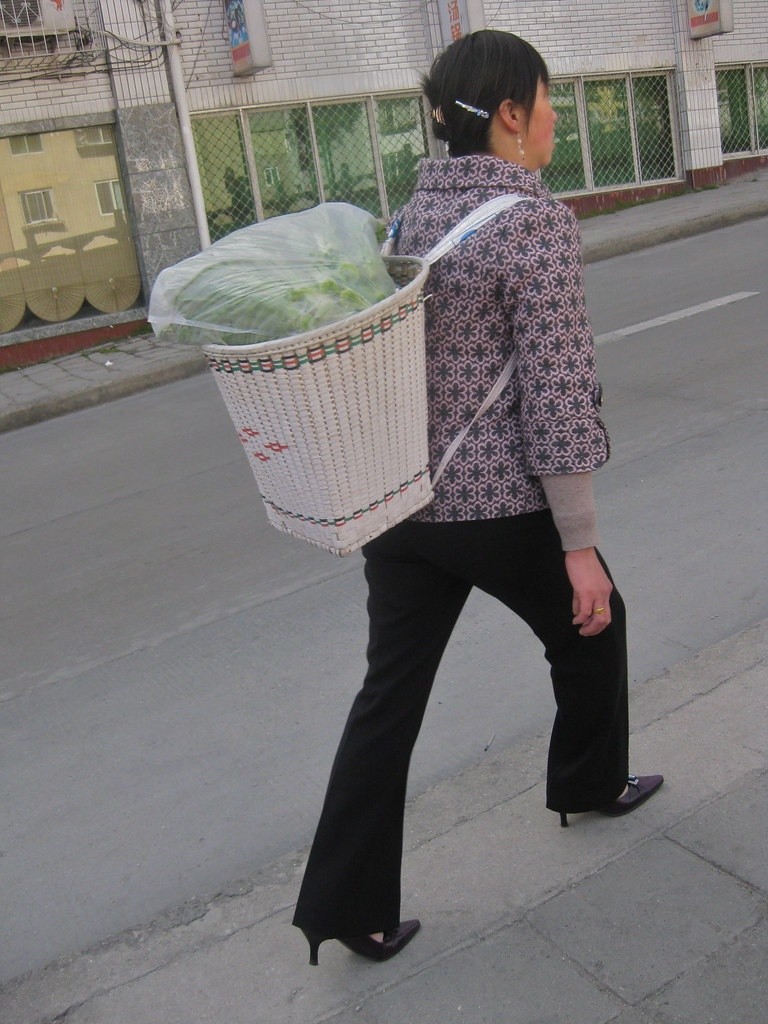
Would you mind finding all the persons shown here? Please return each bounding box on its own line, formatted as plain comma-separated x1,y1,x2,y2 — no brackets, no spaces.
290,29,664,968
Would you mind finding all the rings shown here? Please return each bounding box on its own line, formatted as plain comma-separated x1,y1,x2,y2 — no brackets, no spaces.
593,608,606,615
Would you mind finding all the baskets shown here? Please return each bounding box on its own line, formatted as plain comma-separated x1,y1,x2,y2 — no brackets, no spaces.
201,252,435,559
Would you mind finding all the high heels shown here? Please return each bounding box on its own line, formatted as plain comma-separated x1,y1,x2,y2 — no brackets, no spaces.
559,774,663,827
301,919,423,968
